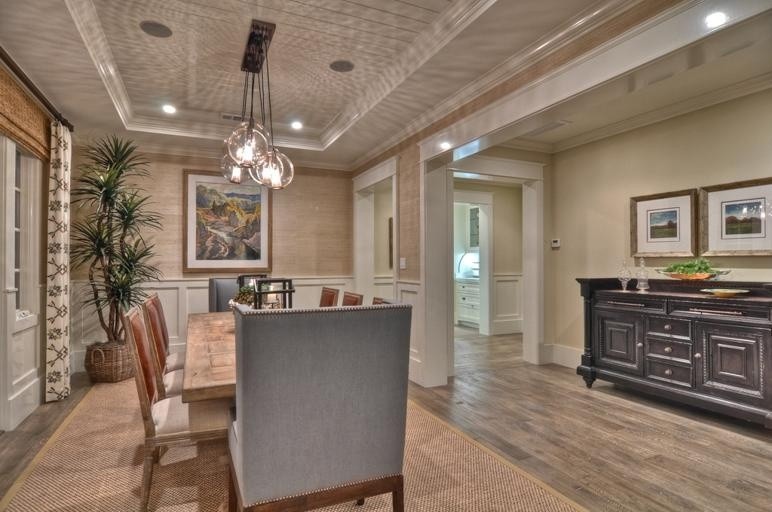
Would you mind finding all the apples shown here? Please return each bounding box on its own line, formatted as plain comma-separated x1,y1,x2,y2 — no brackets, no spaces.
664,260,715,272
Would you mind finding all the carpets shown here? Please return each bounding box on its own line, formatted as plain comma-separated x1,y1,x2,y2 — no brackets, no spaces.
2,377,586,511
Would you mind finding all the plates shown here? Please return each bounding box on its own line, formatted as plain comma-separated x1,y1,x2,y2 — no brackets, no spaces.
701,288,750,298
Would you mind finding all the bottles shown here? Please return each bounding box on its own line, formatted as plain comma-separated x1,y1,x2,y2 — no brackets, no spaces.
618,257,650,293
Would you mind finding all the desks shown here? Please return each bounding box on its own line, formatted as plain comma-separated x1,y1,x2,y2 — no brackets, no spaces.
577,277,772,430
180,310,310,411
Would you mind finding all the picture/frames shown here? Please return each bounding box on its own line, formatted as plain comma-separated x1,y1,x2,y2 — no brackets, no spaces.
628,178,772,259
181,168,272,273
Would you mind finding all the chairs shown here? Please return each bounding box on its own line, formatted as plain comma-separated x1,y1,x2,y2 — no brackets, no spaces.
206,276,270,312
315,286,397,308
123,293,235,511
225,300,413,512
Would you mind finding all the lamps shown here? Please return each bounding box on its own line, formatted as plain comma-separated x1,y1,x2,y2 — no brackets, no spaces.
219,16,294,189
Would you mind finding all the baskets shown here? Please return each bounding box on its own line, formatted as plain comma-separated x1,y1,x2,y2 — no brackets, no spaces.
228,295,281,310
84,343,136,383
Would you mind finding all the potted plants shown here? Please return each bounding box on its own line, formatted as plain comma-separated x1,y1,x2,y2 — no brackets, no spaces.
67,135,165,384
